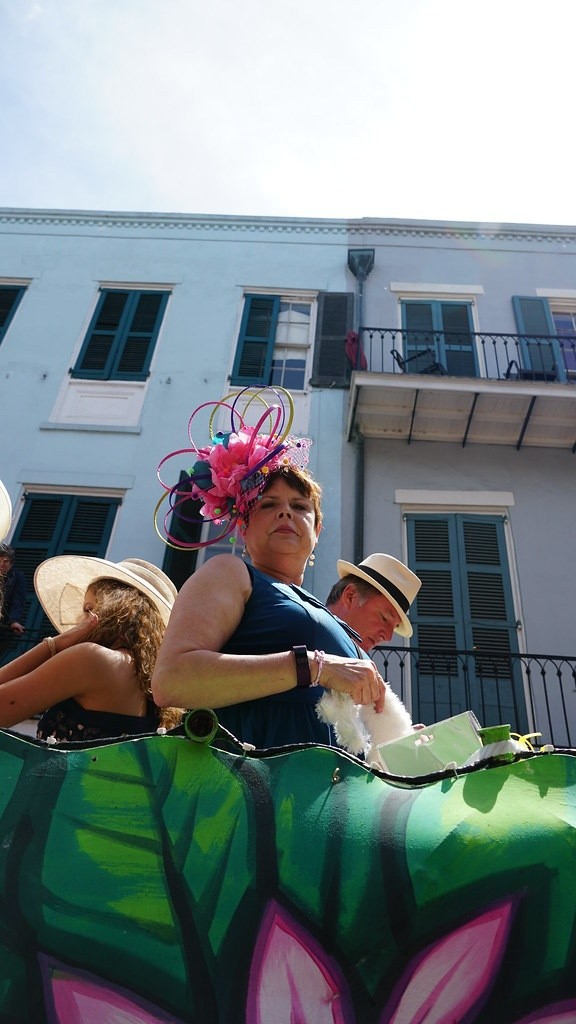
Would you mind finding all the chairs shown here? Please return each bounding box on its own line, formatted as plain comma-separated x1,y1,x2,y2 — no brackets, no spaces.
502,357,563,381
389,347,449,375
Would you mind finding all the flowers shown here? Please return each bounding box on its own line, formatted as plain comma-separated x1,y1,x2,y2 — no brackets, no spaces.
147,381,317,558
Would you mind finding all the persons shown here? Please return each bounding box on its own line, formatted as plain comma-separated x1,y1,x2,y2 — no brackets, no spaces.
0,541,25,654
0,553,178,743
151,464,426,762
321,551,422,650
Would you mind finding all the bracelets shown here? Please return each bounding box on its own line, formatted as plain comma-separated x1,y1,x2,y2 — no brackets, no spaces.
292,645,310,687
310,649,324,688
43,637,56,656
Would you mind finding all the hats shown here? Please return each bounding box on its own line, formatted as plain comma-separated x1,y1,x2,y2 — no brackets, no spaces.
337,553,422,638
0,480,12,543
34,554,178,634
